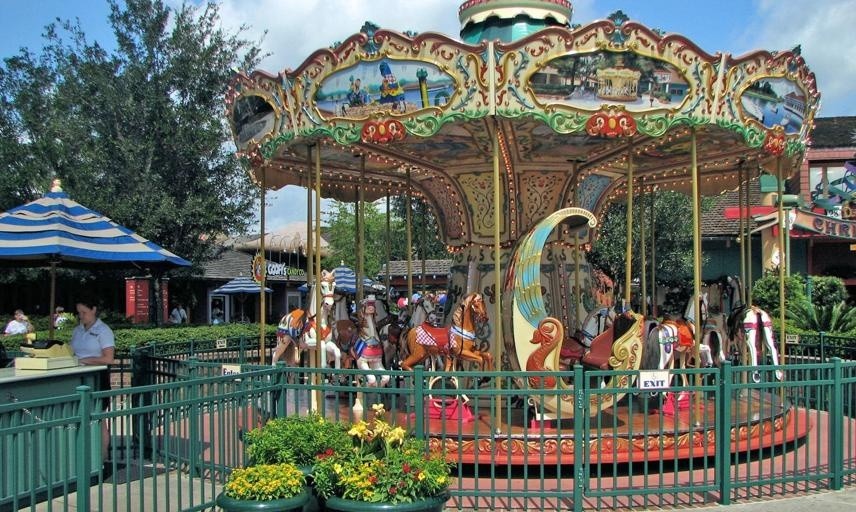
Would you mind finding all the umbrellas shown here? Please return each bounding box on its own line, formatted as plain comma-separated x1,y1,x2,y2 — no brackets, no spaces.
209,271,275,320
0,178,196,341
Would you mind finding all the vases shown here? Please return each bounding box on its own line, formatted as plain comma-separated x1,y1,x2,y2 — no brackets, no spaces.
217,488,451,512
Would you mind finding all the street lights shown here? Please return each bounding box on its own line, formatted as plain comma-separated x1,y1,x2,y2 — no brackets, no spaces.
772,193,804,279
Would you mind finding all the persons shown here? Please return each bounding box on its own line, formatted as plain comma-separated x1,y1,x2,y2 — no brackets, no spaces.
2,308,33,335
69,294,117,474
52,306,64,329
170,302,188,326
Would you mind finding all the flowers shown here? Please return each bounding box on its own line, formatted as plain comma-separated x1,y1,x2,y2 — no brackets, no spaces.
220,402,459,507
